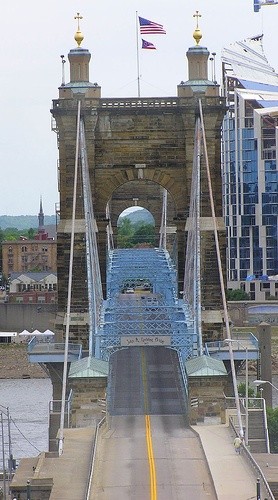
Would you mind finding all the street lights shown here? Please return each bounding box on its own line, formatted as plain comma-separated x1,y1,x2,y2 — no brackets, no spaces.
209,52,217,82
60,54,67,86
222,338,249,446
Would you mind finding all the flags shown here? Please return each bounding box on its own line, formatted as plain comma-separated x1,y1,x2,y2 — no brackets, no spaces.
142,39,156,49
139,16,166,34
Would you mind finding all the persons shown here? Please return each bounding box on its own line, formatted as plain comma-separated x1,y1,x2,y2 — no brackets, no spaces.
234,436,242,455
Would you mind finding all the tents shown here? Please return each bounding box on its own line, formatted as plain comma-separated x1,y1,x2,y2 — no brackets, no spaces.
19,329,55,344
